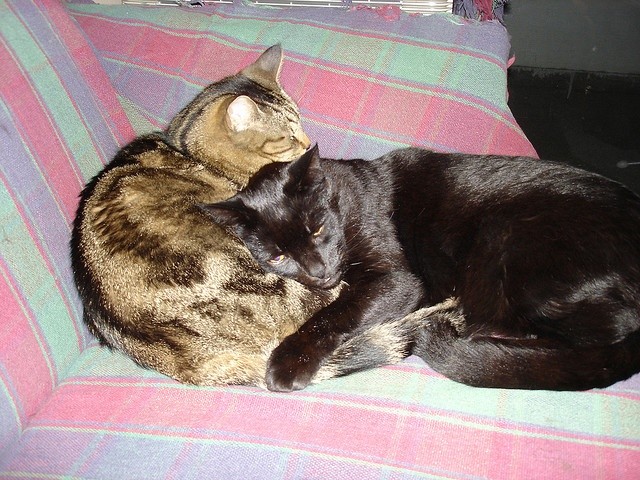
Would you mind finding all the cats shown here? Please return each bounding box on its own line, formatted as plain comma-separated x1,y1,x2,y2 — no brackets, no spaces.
201,140,640,393
68,43,465,390
